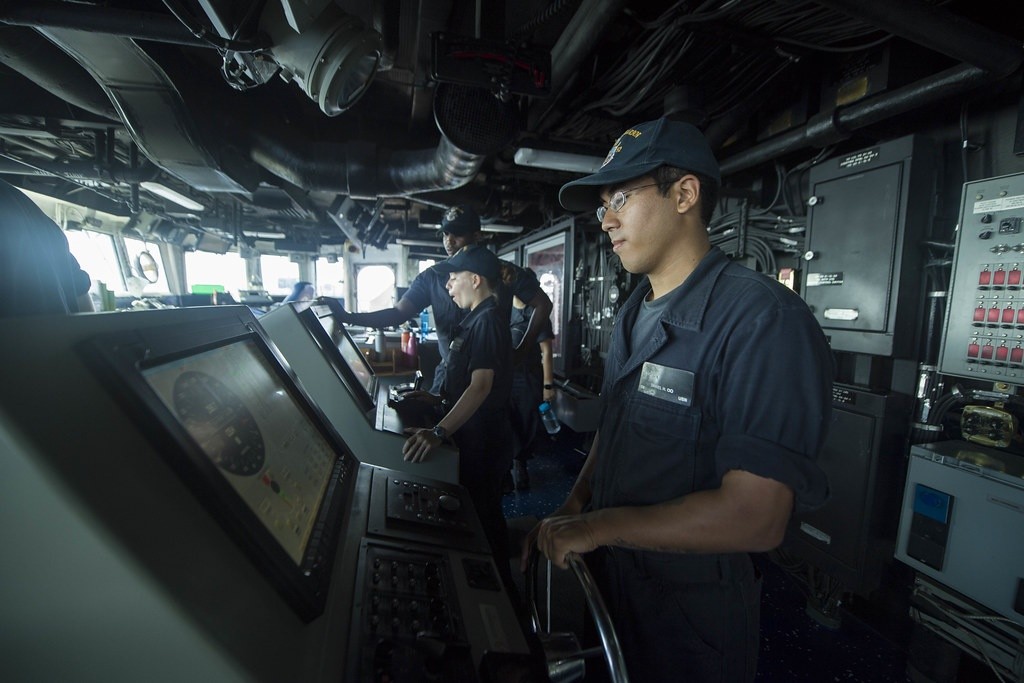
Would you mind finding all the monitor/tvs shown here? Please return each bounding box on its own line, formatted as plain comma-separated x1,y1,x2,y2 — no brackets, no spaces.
141,338,340,565
319,314,372,390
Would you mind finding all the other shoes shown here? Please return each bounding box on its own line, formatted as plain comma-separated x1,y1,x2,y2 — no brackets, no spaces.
513,459,530,489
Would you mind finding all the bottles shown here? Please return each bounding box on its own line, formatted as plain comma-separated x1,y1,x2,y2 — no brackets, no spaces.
539,401,560,434
375,327,386,361
401,326,413,352
420,309,429,335
407,332,417,357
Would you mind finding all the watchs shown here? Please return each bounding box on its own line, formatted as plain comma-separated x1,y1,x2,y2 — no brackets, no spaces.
543,383,554,389
433,425,446,440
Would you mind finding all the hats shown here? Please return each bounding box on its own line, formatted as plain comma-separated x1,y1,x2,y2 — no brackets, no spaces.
431,245,500,282
559,118,722,210
436,205,480,237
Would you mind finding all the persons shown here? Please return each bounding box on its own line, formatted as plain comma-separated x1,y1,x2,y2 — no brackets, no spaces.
400,243,511,493
512,267,555,490
323,206,554,366
520,121,836,683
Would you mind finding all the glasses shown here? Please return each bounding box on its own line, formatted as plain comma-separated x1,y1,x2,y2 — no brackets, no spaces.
596,179,681,222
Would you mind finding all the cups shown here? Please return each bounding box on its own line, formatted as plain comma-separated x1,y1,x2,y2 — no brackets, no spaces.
107,291,115,311
98,284,108,311
